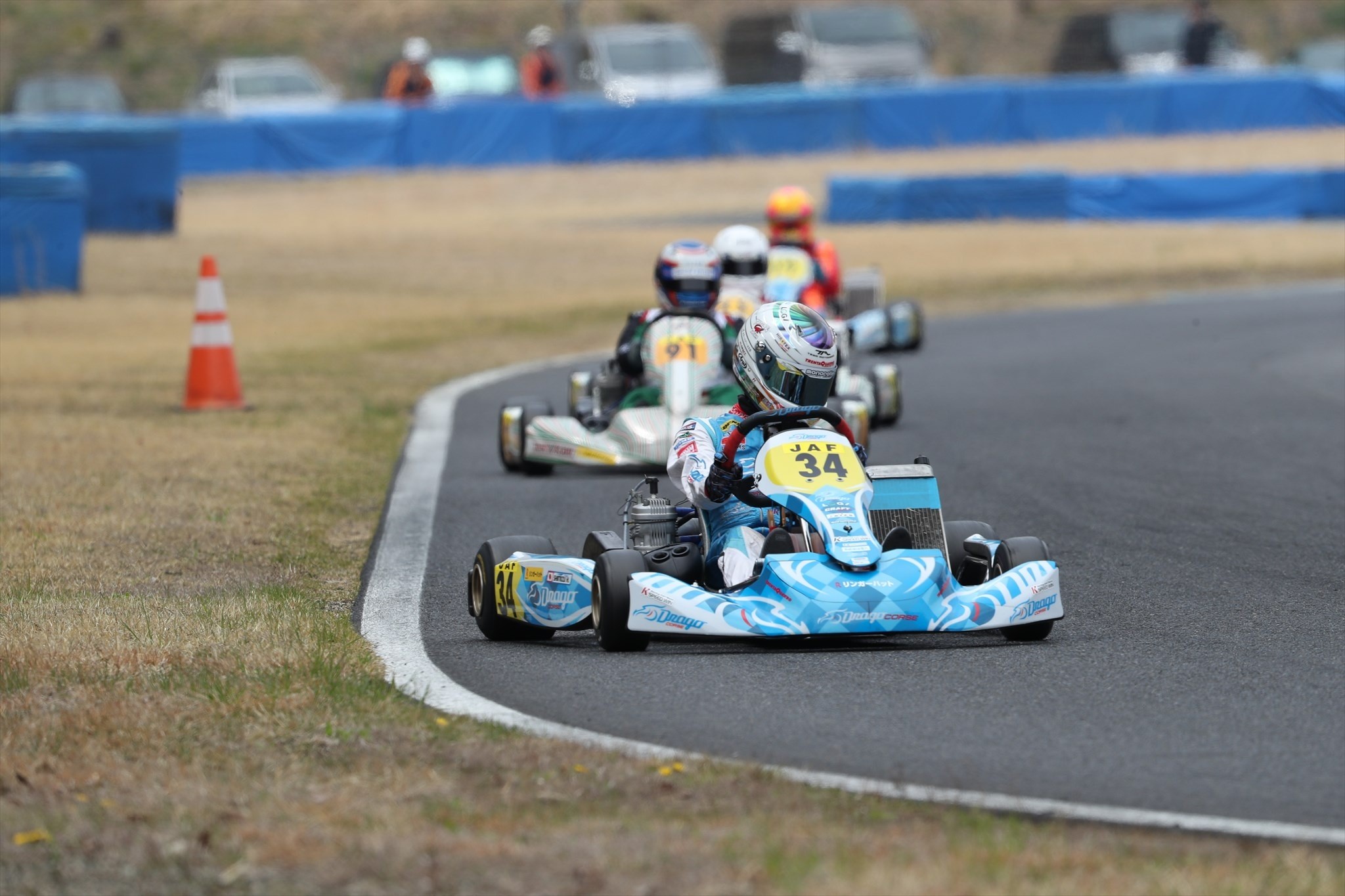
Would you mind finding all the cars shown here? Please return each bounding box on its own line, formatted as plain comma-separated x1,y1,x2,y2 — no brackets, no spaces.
182,55,344,116
1279,29,1345,73
1,69,140,119
370,46,521,104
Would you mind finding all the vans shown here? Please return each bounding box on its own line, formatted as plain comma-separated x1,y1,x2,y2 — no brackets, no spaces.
720,4,931,82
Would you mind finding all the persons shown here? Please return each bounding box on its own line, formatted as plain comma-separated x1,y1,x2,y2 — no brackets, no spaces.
384,36,435,100
667,300,912,591
520,24,566,100
1181,0,1223,66
614,185,844,389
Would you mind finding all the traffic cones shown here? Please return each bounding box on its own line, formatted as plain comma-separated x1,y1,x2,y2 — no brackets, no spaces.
166,256,257,412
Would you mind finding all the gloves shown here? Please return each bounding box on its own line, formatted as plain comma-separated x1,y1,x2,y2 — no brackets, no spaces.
705,453,743,504
853,443,868,467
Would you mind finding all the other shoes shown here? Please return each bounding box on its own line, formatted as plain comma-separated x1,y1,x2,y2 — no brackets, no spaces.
881,527,912,553
754,528,795,575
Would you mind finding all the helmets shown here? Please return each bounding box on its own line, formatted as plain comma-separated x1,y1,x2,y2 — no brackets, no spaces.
733,301,839,427
764,187,816,242
713,225,766,289
653,237,722,314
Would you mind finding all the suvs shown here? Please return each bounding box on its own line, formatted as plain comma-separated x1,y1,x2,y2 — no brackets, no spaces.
1049,7,1265,75
552,21,724,99
